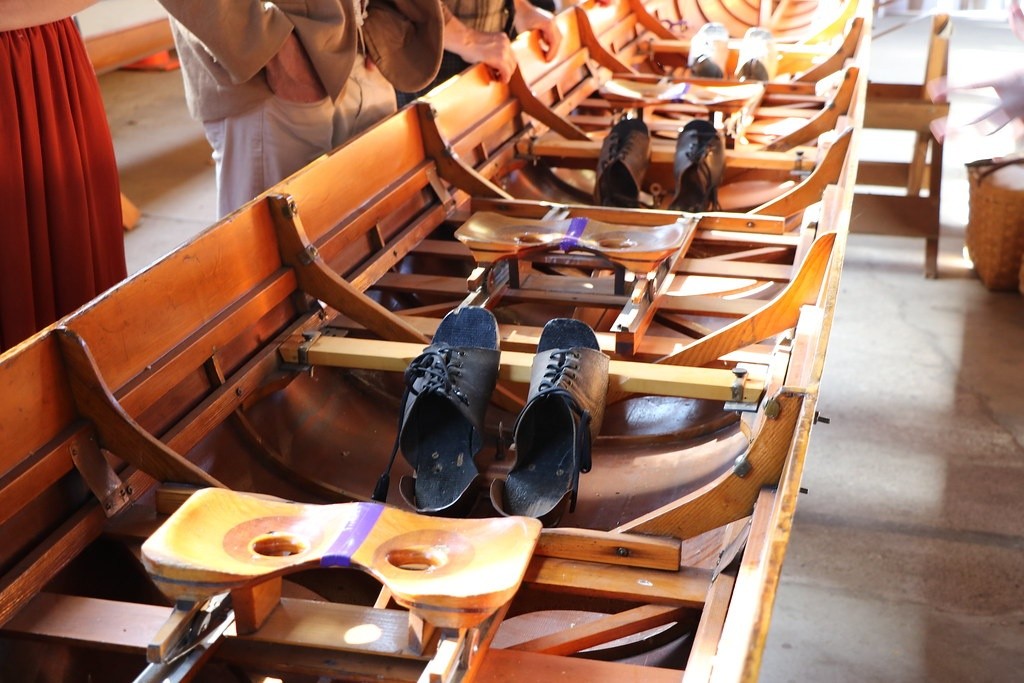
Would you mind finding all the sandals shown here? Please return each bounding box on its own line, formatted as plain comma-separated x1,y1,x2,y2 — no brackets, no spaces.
667,119,725,216
592,119,652,208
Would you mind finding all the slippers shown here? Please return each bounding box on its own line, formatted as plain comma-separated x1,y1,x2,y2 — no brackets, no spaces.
735,25,778,83
688,21,729,80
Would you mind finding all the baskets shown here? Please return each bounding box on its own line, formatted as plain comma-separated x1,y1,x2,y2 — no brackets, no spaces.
963,157,1024,294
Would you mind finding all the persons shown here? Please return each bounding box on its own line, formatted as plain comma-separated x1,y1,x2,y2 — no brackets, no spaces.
394,0,613,109
0,0,127,354
157,0,445,222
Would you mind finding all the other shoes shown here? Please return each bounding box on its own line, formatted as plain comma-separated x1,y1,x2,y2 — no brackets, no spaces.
487,317,611,531
373,304,501,520
